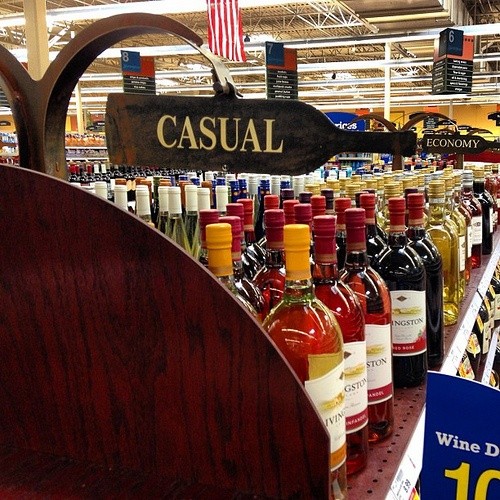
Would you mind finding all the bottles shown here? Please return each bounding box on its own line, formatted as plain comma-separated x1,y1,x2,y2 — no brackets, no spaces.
66,164,500,500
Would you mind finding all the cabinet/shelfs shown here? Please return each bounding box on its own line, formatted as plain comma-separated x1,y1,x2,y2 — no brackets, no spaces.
345,227,500,500
65,146,109,160
